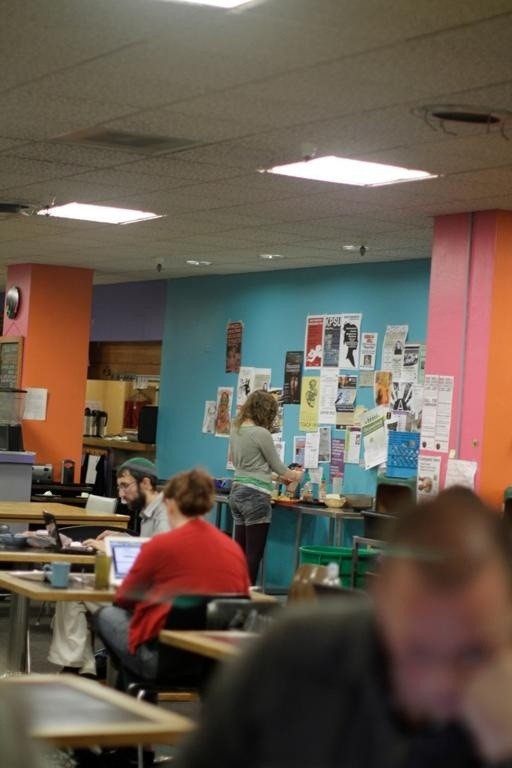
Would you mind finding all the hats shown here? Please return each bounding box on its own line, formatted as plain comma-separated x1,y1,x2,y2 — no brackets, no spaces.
120,457,157,477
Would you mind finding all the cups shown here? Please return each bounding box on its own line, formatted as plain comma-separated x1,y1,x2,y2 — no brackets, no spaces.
113,373,134,381
309,466,322,501
94,555,112,590
43,561,71,588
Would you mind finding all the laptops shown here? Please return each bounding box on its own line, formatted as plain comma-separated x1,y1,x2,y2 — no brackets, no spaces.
42,511,96,555
104,536,151,591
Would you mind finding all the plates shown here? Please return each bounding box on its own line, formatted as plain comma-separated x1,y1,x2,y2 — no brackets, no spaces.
74,496,87,499
273,498,296,504
35,494,59,499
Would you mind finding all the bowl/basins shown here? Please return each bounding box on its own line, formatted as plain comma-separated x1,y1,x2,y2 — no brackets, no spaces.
344,494,373,509
324,496,346,508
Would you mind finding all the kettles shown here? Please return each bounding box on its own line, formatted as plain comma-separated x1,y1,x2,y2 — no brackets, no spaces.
93,411,108,437
83,408,98,436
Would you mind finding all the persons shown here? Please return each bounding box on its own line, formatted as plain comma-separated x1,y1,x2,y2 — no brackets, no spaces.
46,456,173,681
98,465,257,701
167,480,510,766
227,390,304,589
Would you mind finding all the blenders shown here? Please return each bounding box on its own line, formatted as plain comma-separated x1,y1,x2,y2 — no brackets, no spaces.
1,388,27,451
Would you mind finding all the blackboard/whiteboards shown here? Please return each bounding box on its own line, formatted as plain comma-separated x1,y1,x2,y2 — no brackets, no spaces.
0,336,24,427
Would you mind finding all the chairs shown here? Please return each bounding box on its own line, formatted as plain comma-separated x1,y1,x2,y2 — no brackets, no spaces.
349,533,386,589
360,510,399,543
